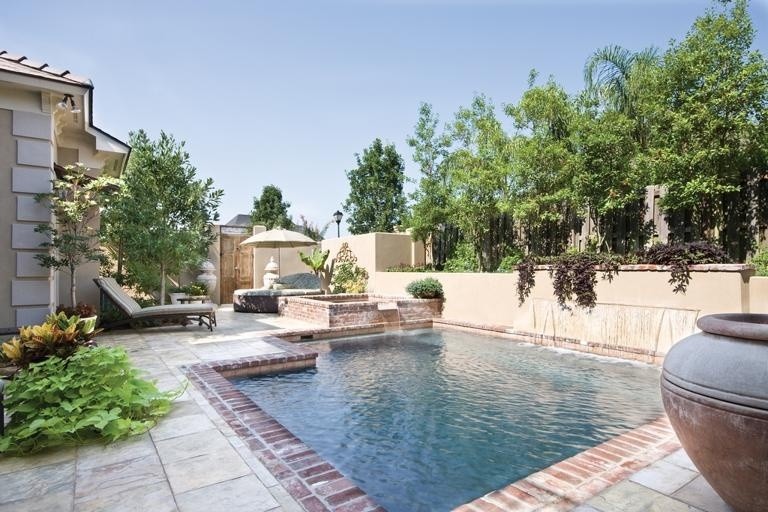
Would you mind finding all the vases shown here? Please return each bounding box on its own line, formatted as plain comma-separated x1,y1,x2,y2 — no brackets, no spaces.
661,313,768,511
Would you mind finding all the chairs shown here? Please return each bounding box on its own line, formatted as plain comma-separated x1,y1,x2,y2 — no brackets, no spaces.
92,277,217,332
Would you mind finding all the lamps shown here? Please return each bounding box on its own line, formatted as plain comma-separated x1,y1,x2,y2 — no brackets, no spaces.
56,94,81,114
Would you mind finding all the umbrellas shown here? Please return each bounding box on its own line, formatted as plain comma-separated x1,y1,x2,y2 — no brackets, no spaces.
240,223,318,283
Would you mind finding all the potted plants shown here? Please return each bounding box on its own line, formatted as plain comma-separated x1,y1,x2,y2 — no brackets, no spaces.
33,161,125,334
183,281,211,304
163,229,200,304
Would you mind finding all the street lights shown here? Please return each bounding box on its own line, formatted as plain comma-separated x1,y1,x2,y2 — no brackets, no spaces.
333,210,343,236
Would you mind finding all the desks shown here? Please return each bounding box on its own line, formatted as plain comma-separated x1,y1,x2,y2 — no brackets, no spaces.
177,298,209,327
